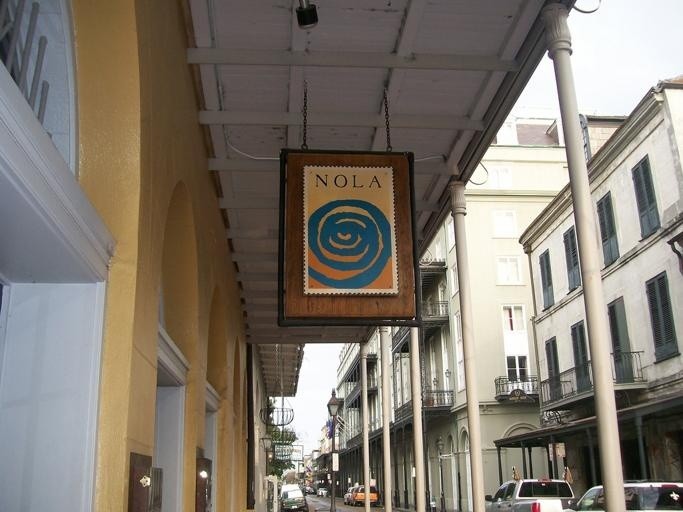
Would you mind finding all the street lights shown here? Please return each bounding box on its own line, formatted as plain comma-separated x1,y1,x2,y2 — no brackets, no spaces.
435,436,446,512
260,433,272,512
327,388,339,512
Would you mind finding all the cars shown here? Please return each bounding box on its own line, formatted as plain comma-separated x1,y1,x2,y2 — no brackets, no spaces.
485,479,683,512
344,485,378,506
280,484,328,512
